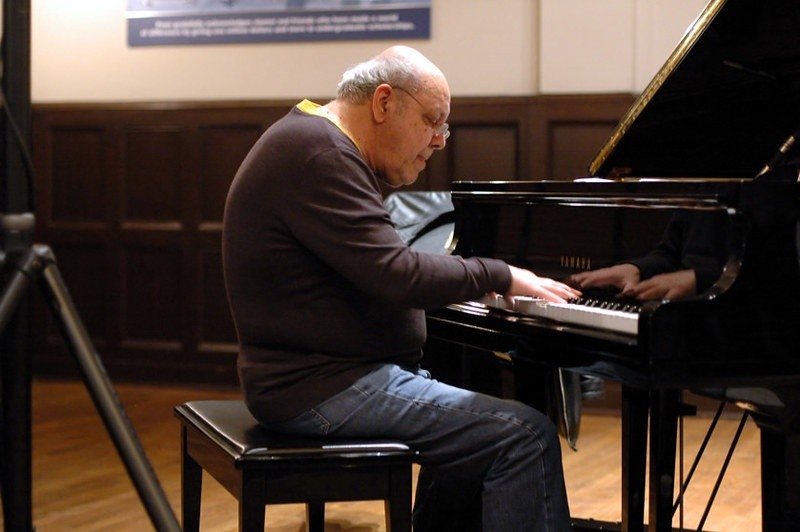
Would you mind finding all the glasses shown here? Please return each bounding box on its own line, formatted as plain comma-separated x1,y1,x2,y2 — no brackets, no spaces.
389,86,450,140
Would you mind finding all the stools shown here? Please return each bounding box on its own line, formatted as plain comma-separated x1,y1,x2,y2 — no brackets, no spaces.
174,399,412,532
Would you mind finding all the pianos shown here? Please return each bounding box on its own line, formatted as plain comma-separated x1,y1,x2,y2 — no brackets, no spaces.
390,0,800,532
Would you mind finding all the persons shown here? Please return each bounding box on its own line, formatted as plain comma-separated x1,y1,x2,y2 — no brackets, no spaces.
221,44,583,532
570,235,716,305
